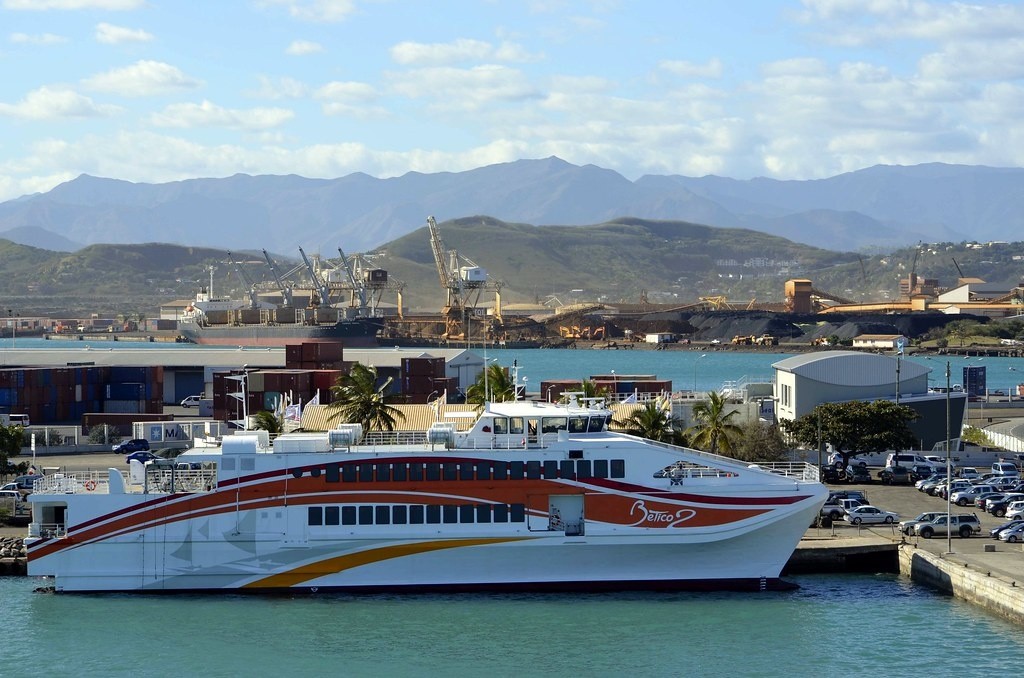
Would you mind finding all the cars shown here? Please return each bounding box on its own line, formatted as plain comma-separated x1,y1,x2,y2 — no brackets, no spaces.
989,519,1024,539
153,447,187,460
712,340,721,344
826,491,863,504
126,450,165,463
843,505,898,525
1005,505,1024,521
998,522,1024,542
911,465,1024,510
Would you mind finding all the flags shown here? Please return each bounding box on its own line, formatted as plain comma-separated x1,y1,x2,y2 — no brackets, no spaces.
654,392,672,414
429,395,448,408
284,405,300,420
620,393,635,404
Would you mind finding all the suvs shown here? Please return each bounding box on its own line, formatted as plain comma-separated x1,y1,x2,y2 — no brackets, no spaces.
923,456,956,470
112,437,149,454
881,466,909,485
896,512,954,536
1006,501,1024,512
914,513,981,538
986,493,1024,517
827,452,870,468
846,465,872,483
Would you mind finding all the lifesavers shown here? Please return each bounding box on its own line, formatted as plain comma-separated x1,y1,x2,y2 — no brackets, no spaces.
85,481,97,492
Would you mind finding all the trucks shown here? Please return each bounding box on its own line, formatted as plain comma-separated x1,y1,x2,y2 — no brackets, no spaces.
679,339,691,345
1001,339,1016,346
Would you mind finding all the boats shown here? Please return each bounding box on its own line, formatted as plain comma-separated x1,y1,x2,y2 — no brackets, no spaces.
25,395,830,593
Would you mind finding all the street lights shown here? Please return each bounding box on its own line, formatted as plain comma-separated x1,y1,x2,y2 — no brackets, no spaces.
925,356,951,553
8,310,20,348
967,358,984,425
694,354,707,391
611,370,616,394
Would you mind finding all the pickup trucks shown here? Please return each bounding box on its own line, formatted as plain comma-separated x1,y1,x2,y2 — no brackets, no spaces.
1,483,33,500
821,499,862,520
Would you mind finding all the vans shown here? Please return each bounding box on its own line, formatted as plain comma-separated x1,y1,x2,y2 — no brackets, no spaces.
9,415,31,427
11,475,42,488
991,463,1020,479
0,491,22,502
886,453,933,472
182,396,200,408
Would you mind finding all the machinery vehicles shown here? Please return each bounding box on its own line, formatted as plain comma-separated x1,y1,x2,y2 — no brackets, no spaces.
731,335,752,345
756,334,779,346
809,338,829,346
560,325,606,341
